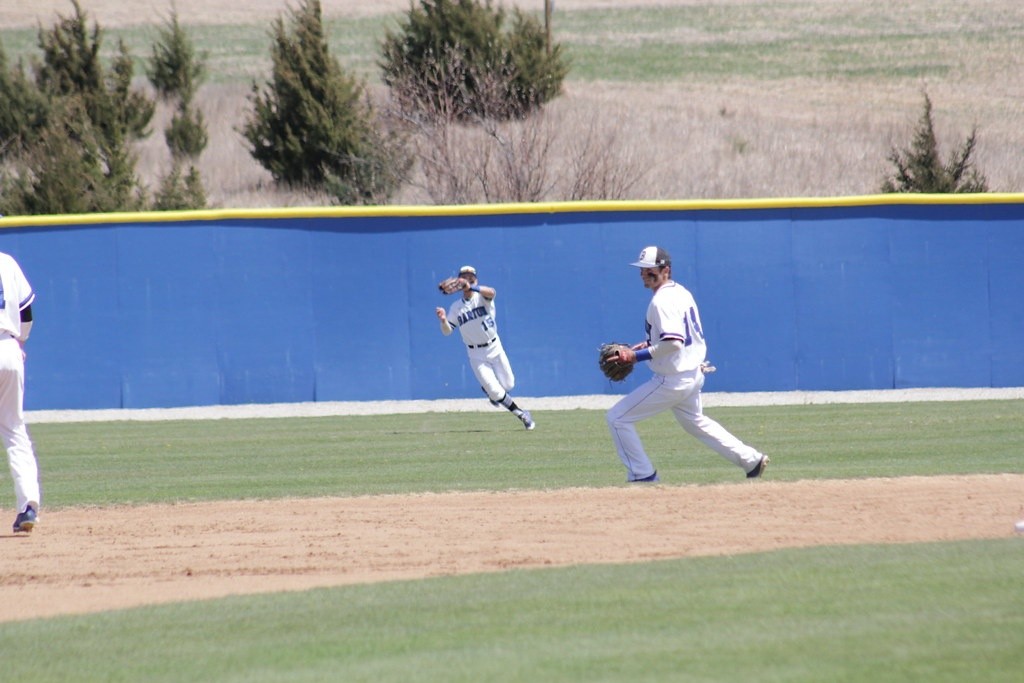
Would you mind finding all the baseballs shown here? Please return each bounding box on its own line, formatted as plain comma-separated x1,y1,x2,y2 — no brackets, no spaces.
439,307,445,312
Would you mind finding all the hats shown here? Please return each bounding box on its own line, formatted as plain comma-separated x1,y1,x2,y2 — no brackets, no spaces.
458,266,477,278
630,246,671,269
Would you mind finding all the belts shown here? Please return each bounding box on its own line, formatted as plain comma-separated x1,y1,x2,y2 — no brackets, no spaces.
468,338,496,348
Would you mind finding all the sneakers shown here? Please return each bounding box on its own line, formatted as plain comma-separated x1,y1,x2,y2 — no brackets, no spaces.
482,386,500,407
747,454,770,479
636,471,660,481
519,410,535,430
13,505,36,532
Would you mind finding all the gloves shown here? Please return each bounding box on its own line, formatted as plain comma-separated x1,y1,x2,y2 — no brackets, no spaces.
701,361,718,374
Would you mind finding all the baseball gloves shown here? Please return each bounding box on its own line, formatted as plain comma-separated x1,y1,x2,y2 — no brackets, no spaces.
595,337,636,389
439,276,466,295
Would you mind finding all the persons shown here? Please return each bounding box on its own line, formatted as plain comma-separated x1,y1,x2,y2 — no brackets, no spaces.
435,265,535,431
0,252,41,533
605,246,770,483
